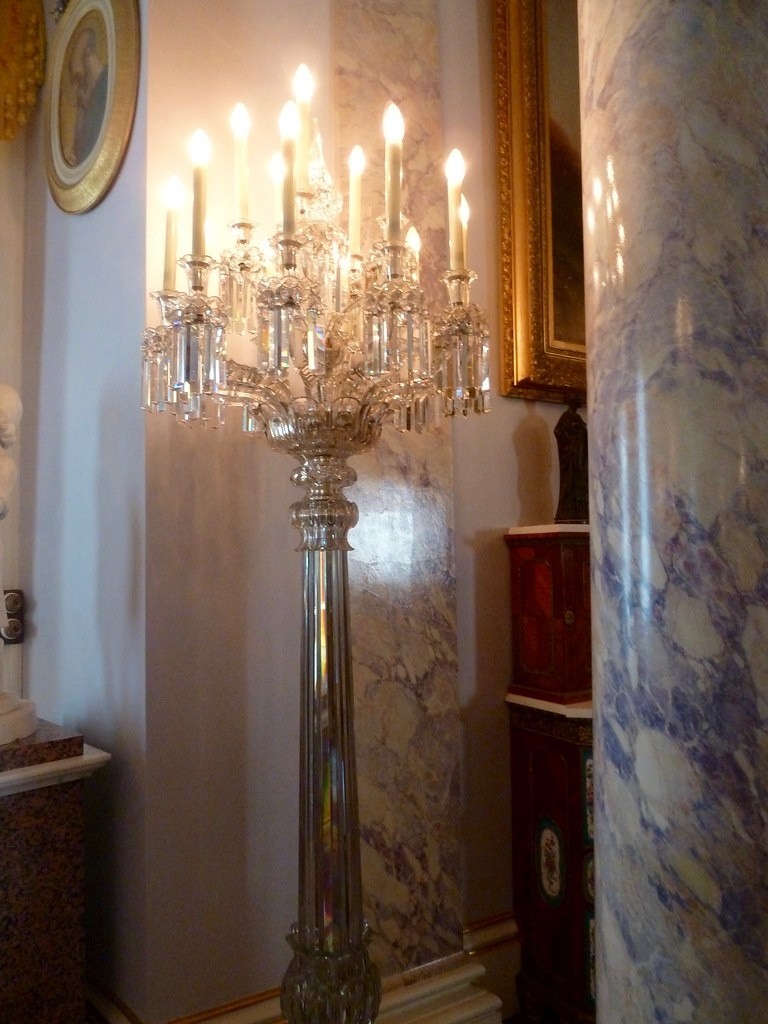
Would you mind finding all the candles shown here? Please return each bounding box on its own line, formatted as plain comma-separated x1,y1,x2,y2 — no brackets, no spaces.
383,102,399,240
277,102,299,232
348,145,364,255
290,62,311,188
461,193,471,272
442,148,462,270
185,128,211,261
228,100,253,222
158,176,183,290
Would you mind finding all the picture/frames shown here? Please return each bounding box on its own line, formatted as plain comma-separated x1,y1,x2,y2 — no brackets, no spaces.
43,0,141,216
490,0,587,406
